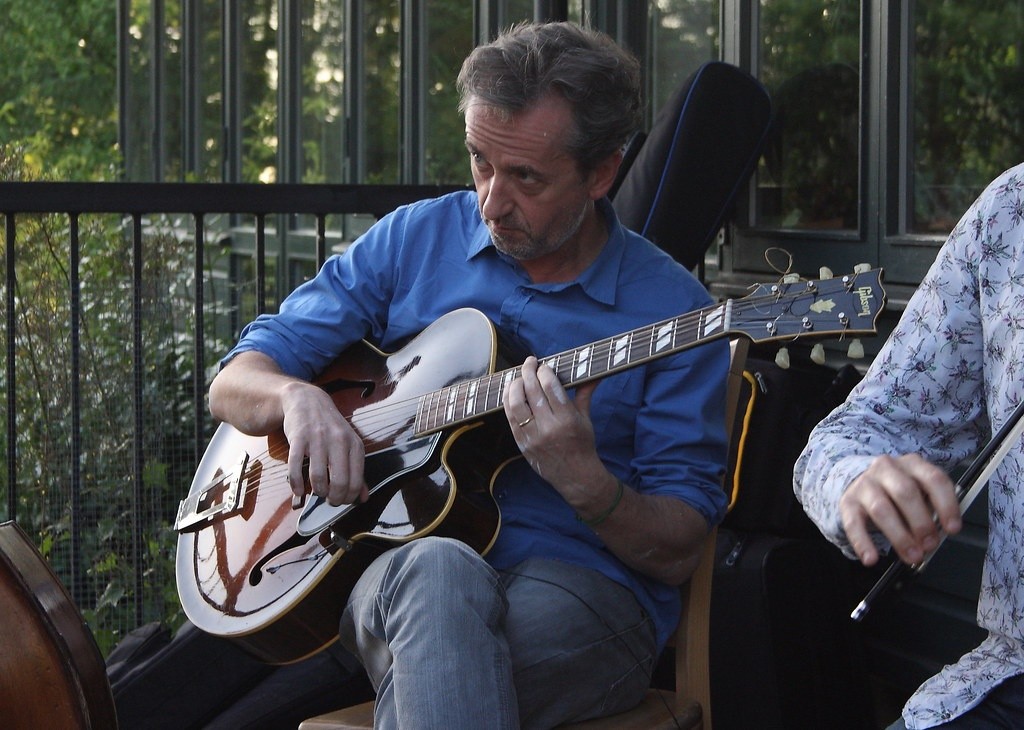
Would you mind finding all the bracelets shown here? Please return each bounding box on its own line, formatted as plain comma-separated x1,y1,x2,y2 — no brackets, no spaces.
574,473,625,526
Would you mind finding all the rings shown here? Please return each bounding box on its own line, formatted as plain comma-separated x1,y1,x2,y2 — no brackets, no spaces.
516,414,536,427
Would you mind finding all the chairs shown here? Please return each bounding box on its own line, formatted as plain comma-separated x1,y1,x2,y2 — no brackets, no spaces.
0,516,119,730
293,329,752,730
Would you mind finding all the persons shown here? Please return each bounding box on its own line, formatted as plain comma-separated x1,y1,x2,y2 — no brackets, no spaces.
209,22,730,730
794,161,1024,730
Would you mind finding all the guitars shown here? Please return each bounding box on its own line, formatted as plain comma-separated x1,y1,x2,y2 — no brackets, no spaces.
174,261,886,664
